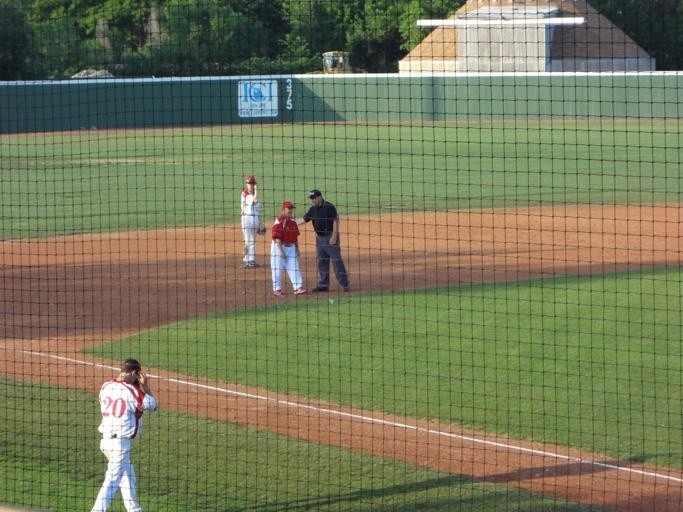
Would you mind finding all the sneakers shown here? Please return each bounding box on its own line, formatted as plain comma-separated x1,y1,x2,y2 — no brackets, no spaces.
292,287,306,295
311,286,351,293
245,261,257,267
273,289,284,296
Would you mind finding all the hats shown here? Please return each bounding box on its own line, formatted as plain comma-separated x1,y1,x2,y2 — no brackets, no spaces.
306,189,322,200
245,175,257,185
282,201,295,210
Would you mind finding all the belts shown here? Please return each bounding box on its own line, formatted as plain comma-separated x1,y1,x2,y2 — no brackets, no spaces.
101,434,117,439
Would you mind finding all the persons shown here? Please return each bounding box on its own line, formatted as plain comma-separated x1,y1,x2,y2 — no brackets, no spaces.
87,357,158,512
269,201,307,296
239,175,261,268
292,189,350,293
